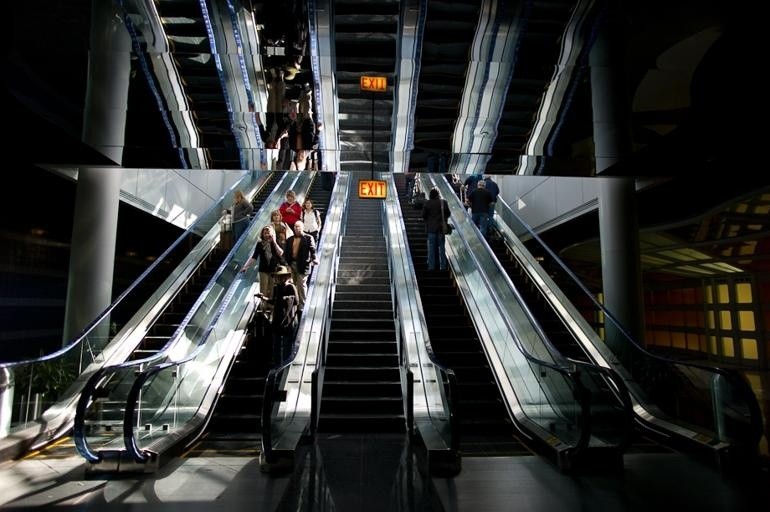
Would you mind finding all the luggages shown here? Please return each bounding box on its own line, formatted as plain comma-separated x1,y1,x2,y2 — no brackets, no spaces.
460,187,468,214
220,209,234,251
253,293,271,338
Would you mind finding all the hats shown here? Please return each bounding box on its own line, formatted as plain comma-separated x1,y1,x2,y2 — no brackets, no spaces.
280,66,301,80
271,265,292,279
477,175,491,189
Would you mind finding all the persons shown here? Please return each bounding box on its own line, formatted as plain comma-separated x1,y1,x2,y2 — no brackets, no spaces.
254,271,300,367
248,0,322,171
218,190,253,248
240,191,322,328
404,173,416,204
460,174,499,236
422,189,451,271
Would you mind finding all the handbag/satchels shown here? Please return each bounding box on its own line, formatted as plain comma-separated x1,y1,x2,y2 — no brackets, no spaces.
442,222,456,235
